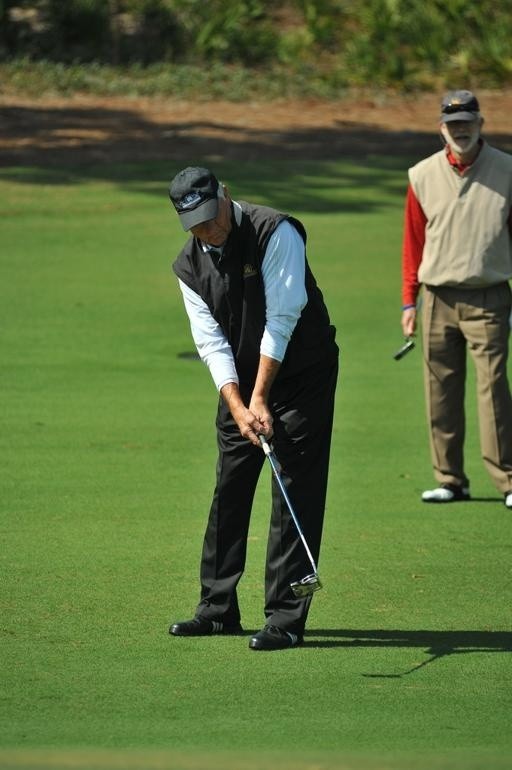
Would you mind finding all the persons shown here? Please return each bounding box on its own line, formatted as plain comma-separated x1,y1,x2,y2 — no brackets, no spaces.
401,90,512,509
169,167,339,649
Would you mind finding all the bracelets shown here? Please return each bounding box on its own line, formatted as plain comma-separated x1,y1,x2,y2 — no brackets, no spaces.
403,304,415,310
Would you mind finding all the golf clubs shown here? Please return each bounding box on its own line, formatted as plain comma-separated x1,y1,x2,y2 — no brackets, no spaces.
392,295,420,362
253,429,321,596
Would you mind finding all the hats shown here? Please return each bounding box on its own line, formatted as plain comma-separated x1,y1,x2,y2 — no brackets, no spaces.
440,92,479,123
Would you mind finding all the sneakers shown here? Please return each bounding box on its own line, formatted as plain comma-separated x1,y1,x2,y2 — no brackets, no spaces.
422,483,470,501
250,625,304,649
168,621,242,636
167,165,219,233
